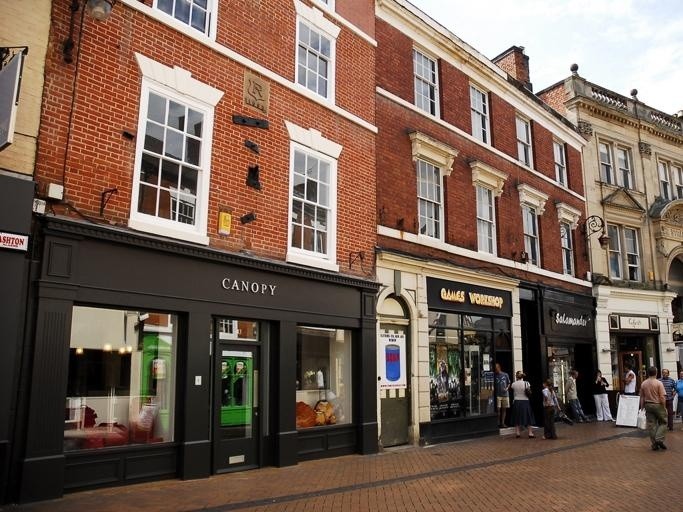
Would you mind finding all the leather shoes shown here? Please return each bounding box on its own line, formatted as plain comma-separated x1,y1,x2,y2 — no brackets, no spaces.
517,434,536,438
652,441,666,450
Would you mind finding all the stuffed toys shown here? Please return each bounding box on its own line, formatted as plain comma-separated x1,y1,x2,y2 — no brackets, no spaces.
313,399,336,425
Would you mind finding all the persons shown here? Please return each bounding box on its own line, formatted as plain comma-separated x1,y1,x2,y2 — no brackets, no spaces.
541,378,562,440
620,362,636,395
671,368,682,423
639,366,668,451
494,362,511,428
656,368,677,431
590,370,613,421
510,371,536,438
564,370,588,423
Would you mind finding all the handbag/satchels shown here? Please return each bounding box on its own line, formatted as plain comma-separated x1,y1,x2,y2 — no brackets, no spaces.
526,390,532,397
